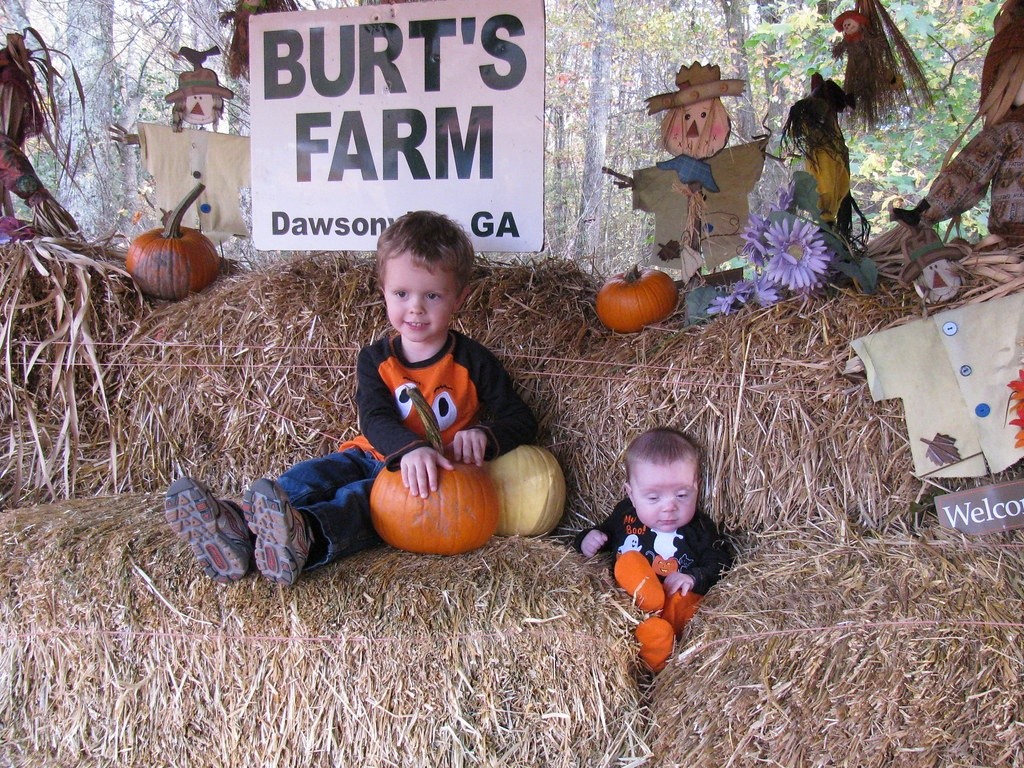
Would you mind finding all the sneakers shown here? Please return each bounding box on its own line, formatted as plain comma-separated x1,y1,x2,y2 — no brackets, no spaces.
243,478,312,586
164,476,254,584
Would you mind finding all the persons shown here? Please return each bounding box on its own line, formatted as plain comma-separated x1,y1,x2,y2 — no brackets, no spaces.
219,0,277,77
109,68,251,247
888,0,1024,240
782,70,854,234
165,212,534,588
846,227,1024,477
576,427,734,674
831,10,896,111
601,60,785,282
0,33,46,249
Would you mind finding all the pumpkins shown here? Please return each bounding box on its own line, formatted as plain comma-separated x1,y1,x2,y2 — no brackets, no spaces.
481,443,565,537
125,183,220,300
596,264,678,334
370,387,500,556
